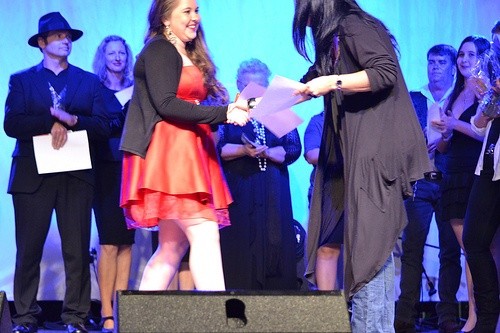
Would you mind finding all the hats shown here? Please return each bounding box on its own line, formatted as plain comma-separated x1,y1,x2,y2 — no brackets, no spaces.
28,11,83,48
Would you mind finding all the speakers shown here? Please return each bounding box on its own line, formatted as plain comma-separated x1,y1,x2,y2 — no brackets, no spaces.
113,290,353,333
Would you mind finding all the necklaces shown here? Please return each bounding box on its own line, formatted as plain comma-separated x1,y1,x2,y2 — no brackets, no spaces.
461,90,475,104
249,119,268,171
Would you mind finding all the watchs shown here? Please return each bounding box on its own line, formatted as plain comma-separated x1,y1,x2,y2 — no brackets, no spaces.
247,97,257,110
336,75,343,91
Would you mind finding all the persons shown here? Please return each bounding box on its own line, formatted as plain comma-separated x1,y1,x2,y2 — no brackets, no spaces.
426,35,493,333
3,11,126,333
393,44,462,333
303,111,343,293
461,21,500,333
229,0,433,333
92,35,135,333
216,58,302,291
118,0,251,291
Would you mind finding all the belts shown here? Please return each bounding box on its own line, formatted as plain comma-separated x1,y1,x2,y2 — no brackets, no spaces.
424,172,442,179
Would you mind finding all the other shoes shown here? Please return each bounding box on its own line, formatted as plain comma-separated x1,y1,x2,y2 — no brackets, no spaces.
100,316,114,333
11,324,37,333
438,321,458,333
394,320,416,333
67,324,88,333
